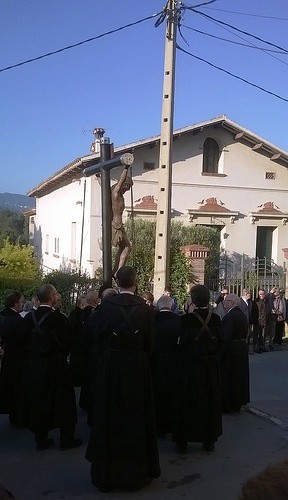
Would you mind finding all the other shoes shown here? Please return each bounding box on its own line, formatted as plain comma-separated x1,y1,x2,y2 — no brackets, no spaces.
60,439,82,450
202,441,215,452
37,439,54,451
179,439,189,454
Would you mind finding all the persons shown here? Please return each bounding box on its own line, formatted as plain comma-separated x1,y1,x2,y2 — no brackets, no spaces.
0,285,250,453
111,165,133,278
239,287,288,355
77,266,161,493
